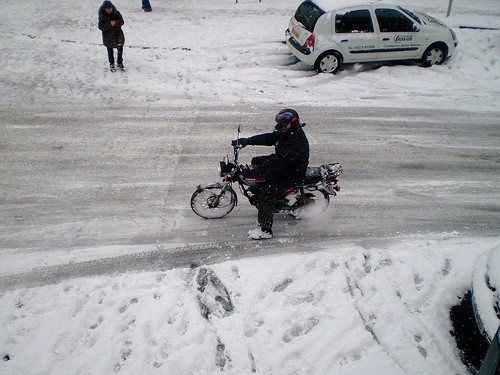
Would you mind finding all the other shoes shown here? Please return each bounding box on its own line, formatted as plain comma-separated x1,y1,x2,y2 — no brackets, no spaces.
117,63,124,71
110,64,116,72
248,226,273,240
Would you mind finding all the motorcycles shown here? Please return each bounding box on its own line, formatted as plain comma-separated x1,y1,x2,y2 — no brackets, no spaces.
190,124,342,221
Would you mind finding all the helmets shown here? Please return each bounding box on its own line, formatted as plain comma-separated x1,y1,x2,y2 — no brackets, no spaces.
274,109,300,136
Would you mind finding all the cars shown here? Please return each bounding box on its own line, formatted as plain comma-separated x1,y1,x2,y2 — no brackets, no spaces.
283,0,459,73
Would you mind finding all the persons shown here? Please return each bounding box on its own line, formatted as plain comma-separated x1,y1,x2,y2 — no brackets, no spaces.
97,0,125,72
234,109,309,239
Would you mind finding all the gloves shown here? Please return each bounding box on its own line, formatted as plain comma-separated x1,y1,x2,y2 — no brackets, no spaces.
231,138,248,148
242,165,260,180
